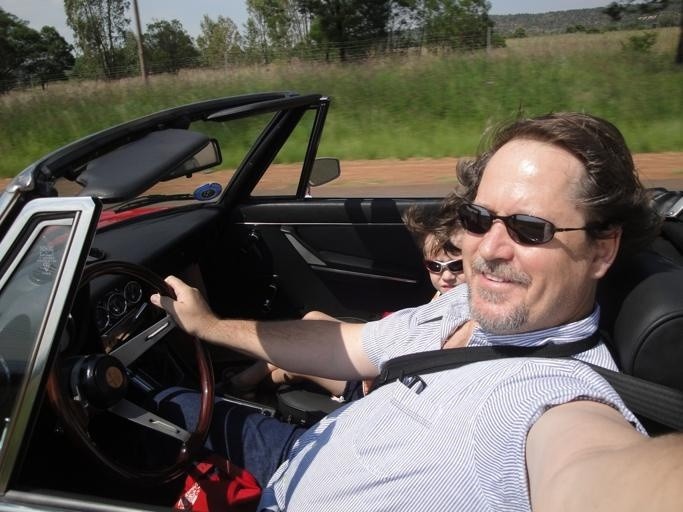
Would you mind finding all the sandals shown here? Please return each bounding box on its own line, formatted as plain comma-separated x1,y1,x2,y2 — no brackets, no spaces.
214,368,259,401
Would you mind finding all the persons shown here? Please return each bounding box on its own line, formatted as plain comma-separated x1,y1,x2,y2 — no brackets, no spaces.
148,106,680,512
214,195,466,403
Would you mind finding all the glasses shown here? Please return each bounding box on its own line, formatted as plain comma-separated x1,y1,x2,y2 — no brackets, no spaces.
457,204,604,245
424,259,463,274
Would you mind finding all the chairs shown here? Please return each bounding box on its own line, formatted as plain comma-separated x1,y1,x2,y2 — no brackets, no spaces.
276,317,373,426
601,237,683,437
